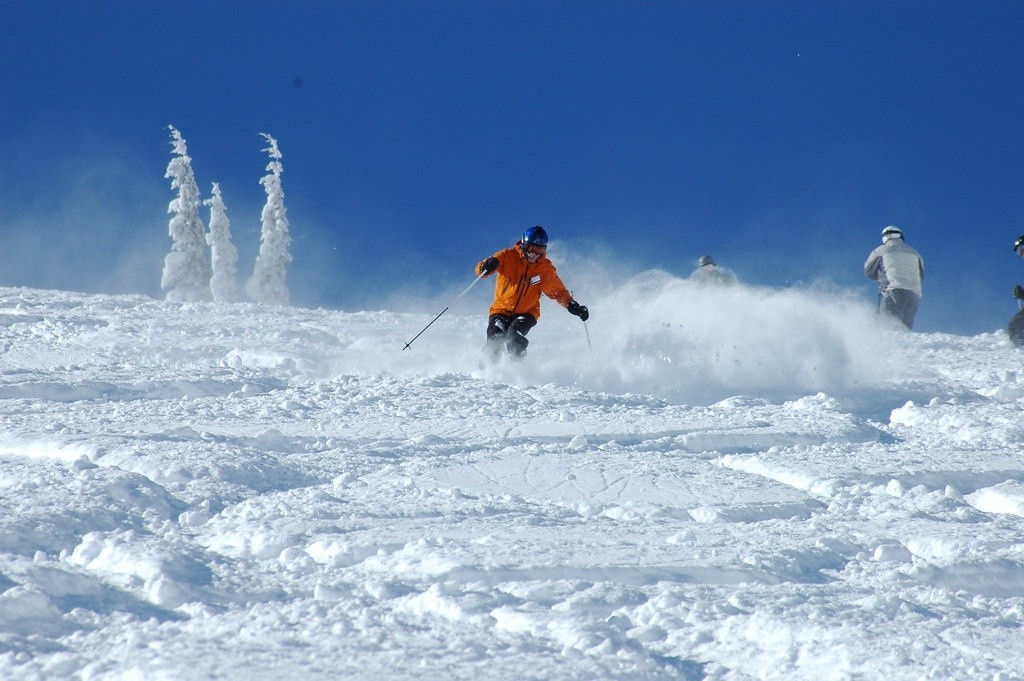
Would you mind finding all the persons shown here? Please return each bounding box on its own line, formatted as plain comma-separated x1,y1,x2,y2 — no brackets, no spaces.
688,255,738,288
475,225,589,363
863,225,925,331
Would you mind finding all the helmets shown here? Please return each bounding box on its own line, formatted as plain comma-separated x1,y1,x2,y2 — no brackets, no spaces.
521,226,548,246
1014,236,1024,250
881,226,903,244
699,255,712,266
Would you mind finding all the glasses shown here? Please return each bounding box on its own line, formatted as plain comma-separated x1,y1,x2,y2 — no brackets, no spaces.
525,243,546,255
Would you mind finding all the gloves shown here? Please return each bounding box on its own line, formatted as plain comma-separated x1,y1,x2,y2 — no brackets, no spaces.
481,258,499,275
567,300,589,321
1012,286,1024,299
